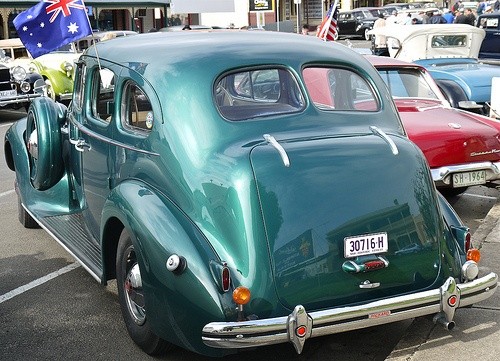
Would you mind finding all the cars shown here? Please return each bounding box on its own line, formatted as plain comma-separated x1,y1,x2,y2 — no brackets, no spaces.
4,29,498,357
0,26,214,121
236,54,500,206
337,0,500,115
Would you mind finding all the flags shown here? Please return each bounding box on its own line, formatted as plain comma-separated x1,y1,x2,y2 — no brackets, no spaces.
317,1,339,42
12,0,92,60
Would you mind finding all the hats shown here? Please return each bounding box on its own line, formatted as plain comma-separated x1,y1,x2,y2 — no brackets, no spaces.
182,25,191,30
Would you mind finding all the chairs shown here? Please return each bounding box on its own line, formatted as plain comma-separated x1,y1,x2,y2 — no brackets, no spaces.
220,103,297,120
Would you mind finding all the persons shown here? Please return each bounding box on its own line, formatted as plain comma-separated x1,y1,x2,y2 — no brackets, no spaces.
301,25,310,36
373,0,500,47
